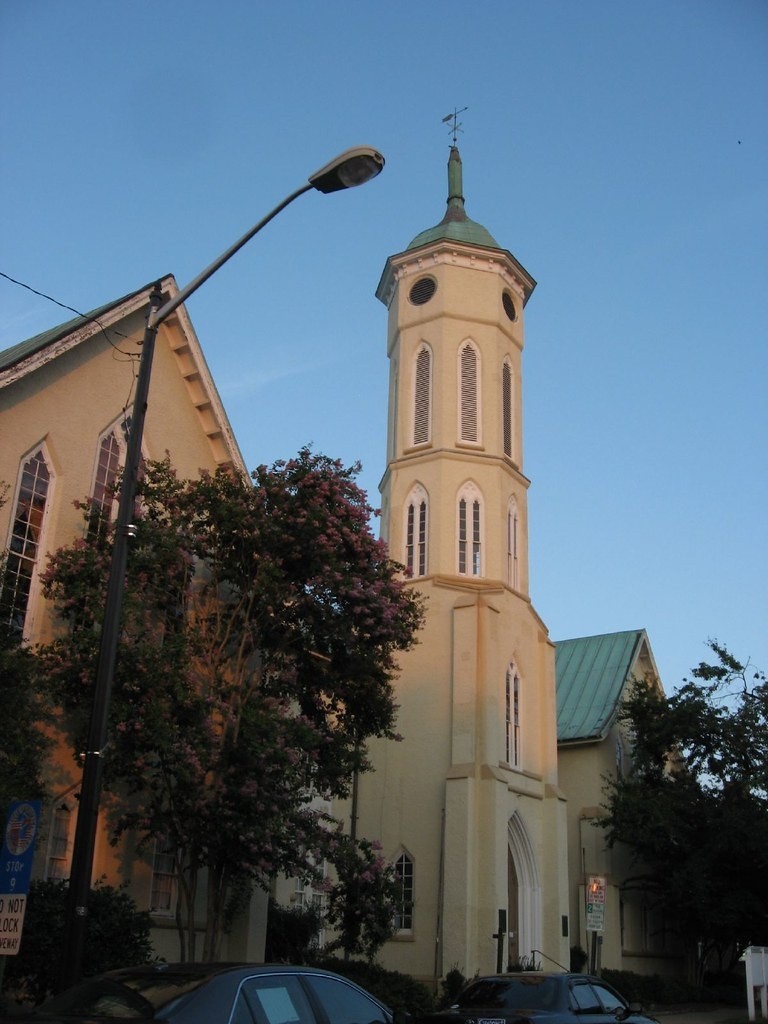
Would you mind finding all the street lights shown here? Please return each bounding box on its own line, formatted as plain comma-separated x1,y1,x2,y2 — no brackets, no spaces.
56,146,387,990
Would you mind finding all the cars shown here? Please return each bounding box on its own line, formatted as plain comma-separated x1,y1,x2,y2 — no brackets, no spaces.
6,963,449,1024
422,970,662,1024
